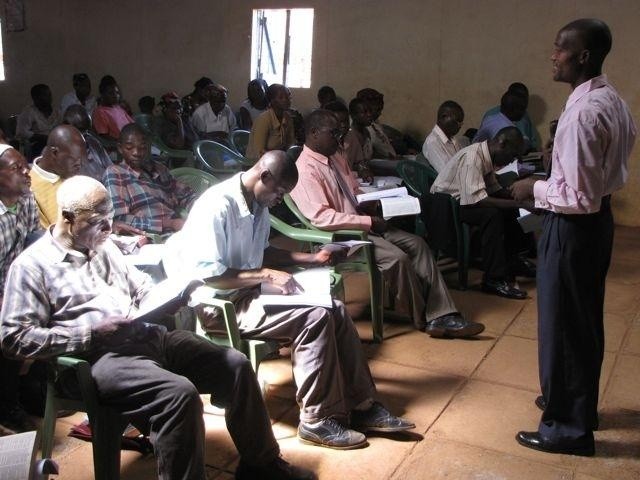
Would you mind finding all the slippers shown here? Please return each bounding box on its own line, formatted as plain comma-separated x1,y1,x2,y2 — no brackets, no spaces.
424,312,485,339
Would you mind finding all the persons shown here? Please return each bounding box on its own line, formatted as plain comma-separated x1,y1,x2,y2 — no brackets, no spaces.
505,18,637,457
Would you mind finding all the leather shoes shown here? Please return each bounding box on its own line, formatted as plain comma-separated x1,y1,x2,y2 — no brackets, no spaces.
347,402,418,434
514,430,595,459
494,283,528,300
534,395,548,411
296,417,368,452
235,456,318,480
516,259,538,280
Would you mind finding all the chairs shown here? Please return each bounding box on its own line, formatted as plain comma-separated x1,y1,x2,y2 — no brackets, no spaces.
28,354,156,480
138,113,267,228
187,156,471,379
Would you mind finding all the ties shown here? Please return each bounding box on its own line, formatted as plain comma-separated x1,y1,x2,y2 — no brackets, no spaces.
328,156,367,218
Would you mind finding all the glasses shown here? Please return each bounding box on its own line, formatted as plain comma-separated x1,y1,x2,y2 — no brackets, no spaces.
313,127,345,142
